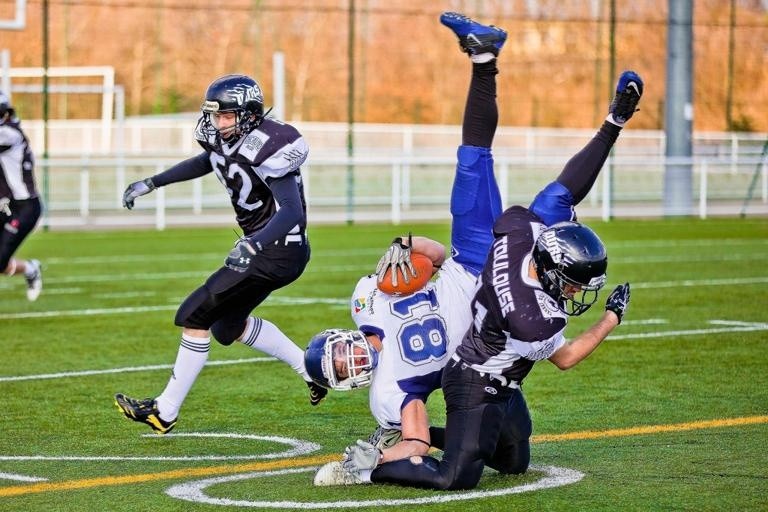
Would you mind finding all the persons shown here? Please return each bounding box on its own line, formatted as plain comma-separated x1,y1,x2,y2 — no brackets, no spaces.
313,206,629,489
115,75,326,433
0,94,42,302
306,11,644,472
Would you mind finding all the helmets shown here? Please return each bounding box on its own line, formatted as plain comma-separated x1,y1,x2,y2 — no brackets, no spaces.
304,328,378,391
200,74,264,147
532,221,609,316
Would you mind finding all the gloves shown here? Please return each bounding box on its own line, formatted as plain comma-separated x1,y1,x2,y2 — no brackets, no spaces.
375,235,417,290
605,282,630,327
122,177,155,210
225,238,264,275
343,439,381,474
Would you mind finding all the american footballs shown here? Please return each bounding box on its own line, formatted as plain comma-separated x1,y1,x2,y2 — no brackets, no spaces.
377,252,433,296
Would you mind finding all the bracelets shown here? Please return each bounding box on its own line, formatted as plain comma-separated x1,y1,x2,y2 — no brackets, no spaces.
379,449,384,464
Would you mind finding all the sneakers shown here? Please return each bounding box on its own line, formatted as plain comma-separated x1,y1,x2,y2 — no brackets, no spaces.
115,393,178,435
24,259,43,302
367,426,403,451
313,461,365,488
609,70,644,125
306,381,328,407
440,12,508,58
